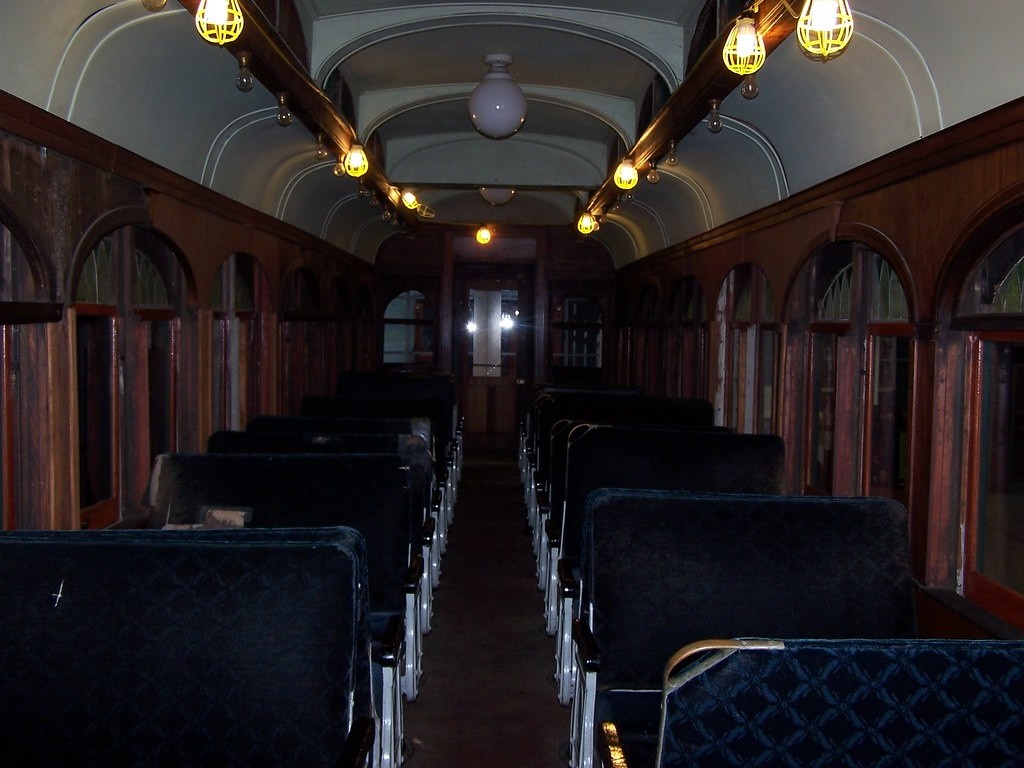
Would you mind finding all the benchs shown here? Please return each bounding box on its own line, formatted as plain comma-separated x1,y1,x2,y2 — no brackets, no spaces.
517,380,1024,768
0,365,469,768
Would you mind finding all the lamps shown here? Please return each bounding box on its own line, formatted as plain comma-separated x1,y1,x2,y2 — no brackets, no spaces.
722,10,766,75
476,223,491,244
478,184,516,205
796,0,853,57
344,142,369,178
614,156,638,189
469,53,528,139
195,0,244,46
401,190,437,219
577,210,596,235
